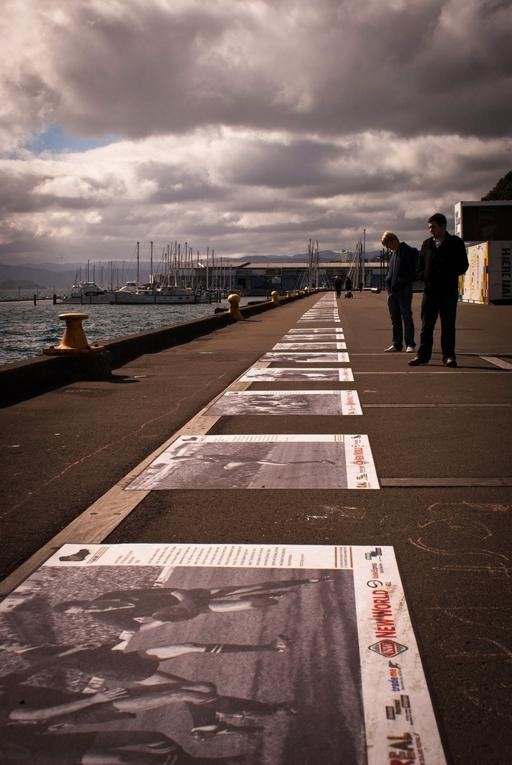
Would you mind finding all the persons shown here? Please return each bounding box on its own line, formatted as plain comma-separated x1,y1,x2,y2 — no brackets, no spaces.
358,280,364,293
334,275,342,298
380,227,422,353
345,276,353,292
408,212,470,366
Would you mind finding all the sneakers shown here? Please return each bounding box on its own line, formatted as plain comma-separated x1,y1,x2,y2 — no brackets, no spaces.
407,355,430,366
384,344,402,352
405,346,415,352
443,357,457,367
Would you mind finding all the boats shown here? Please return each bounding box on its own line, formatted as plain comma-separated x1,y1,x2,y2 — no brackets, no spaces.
61,286,228,305
115,281,142,294
71,281,106,297
